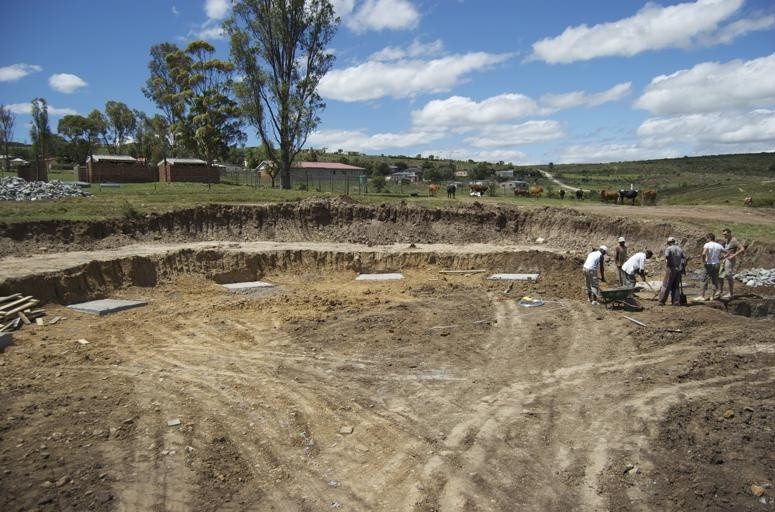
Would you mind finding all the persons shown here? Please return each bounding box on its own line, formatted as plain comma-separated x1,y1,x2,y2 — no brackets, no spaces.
559,188,565,200
580,187,583,199
716,228,745,297
657,237,692,306
622,250,653,301
583,245,608,306
614,237,628,284
693,233,729,300
576,187,580,199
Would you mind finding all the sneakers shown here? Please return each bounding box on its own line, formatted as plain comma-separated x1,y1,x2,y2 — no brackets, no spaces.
693,292,734,301
588,297,600,305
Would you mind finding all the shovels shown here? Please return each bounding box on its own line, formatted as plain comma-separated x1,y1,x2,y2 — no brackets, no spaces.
679,269,687,304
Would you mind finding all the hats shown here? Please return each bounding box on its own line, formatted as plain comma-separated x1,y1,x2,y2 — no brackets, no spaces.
668,237,675,242
618,237,625,242
600,245,608,253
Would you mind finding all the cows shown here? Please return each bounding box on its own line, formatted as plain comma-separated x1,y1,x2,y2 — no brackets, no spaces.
429,184,441,197
619,190,639,205
468,184,488,196
640,189,656,204
528,186,544,199
601,190,621,204
447,184,456,198
513,187,528,197
743,197,752,207
576,191,579,198
